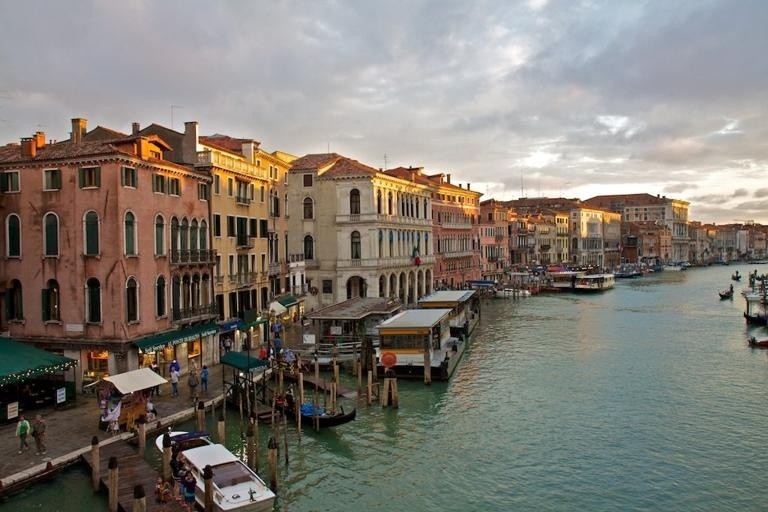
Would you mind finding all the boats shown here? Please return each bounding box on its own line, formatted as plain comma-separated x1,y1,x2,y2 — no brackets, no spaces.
302,428,358,466
270,391,357,427
468,256,693,298
748,260,768,264
155,424,276,511
748,336,768,347
730,273,741,282
305,286,480,384
743,310,767,326
718,284,735,299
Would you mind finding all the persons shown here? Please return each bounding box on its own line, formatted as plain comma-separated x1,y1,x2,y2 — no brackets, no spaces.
223,321,282,362
30,414,47,456
729,269,768,298
154,446,197,510
292,311,304,326
286,384,294,411
146,359,209,416
15,415,30,454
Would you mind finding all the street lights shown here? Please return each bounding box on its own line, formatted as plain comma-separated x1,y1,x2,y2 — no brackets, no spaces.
238,304,260,379
263,302,287,362
